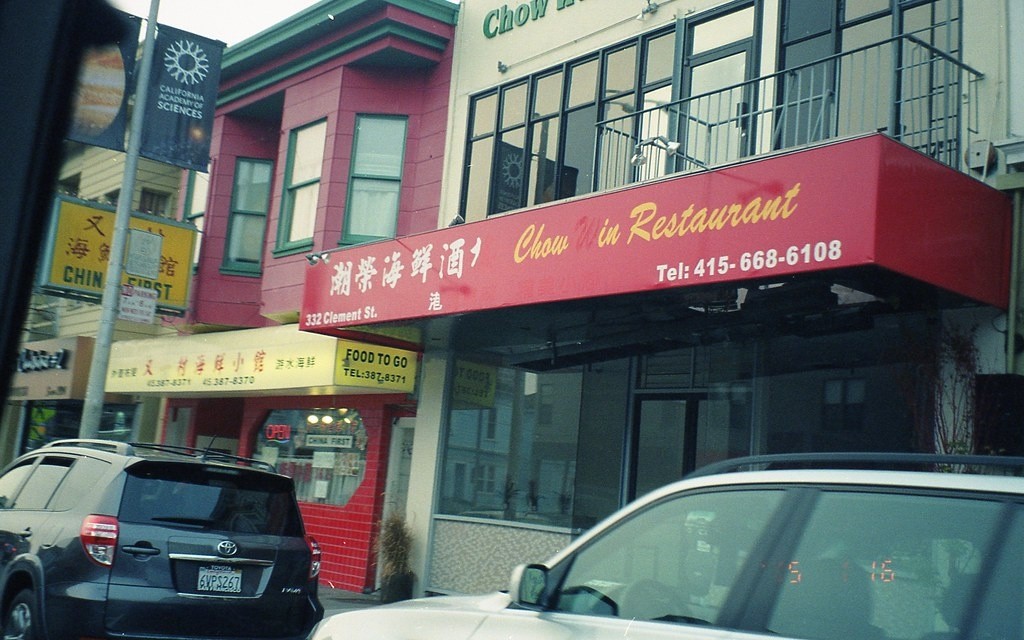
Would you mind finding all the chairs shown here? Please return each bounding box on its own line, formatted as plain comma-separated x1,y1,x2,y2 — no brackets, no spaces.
768,558,886,640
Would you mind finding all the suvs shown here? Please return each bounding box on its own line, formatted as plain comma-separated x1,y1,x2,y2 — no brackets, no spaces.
302,452,1024,640
0,438,325,640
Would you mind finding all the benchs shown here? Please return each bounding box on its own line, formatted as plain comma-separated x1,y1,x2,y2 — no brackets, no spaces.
919,573,982,639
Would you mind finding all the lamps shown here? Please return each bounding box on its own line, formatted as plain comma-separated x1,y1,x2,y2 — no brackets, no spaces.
630,136,711,173
322,410,333,424
306,244,331,265
344,411,358,423
636,0,659,21
